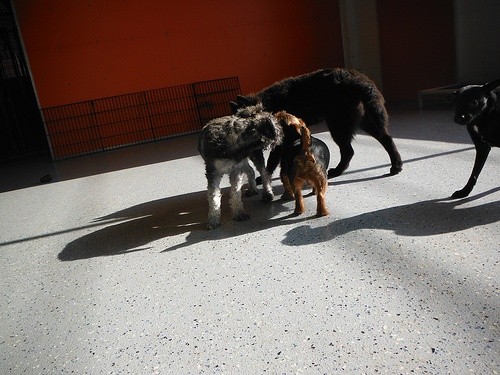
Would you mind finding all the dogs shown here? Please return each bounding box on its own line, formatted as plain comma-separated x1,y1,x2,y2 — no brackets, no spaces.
450,80,500,199
195,66,404,230
